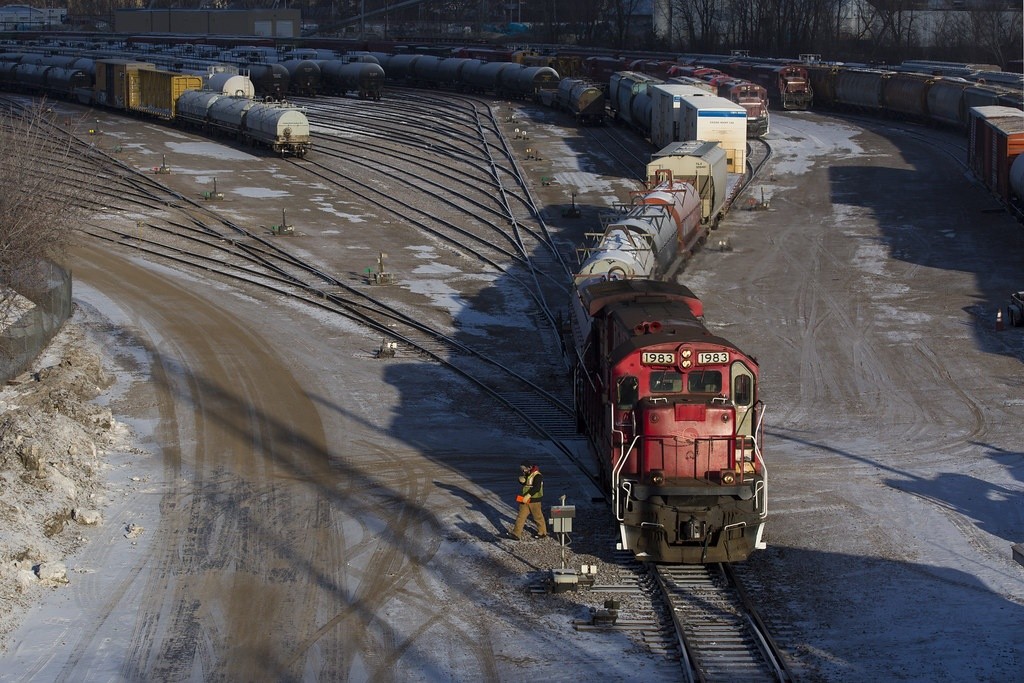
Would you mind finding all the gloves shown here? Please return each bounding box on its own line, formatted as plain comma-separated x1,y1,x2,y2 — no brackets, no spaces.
520,478,526,483
522,493,531,503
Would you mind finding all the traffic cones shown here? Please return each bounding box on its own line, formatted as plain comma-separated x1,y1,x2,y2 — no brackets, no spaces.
995,309,1006,331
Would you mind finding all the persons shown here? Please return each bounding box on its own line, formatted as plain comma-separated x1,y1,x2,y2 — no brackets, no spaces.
506,460,547,541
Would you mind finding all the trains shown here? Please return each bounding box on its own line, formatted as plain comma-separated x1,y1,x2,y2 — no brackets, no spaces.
672,49,1024,266
570,69,769,564
0,31,813,162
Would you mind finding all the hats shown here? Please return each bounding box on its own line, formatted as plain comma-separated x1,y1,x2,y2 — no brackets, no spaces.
520,460,530,473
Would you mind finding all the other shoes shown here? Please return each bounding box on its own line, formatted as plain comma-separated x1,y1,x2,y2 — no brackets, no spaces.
535,534,546,539
505,533,521,542
